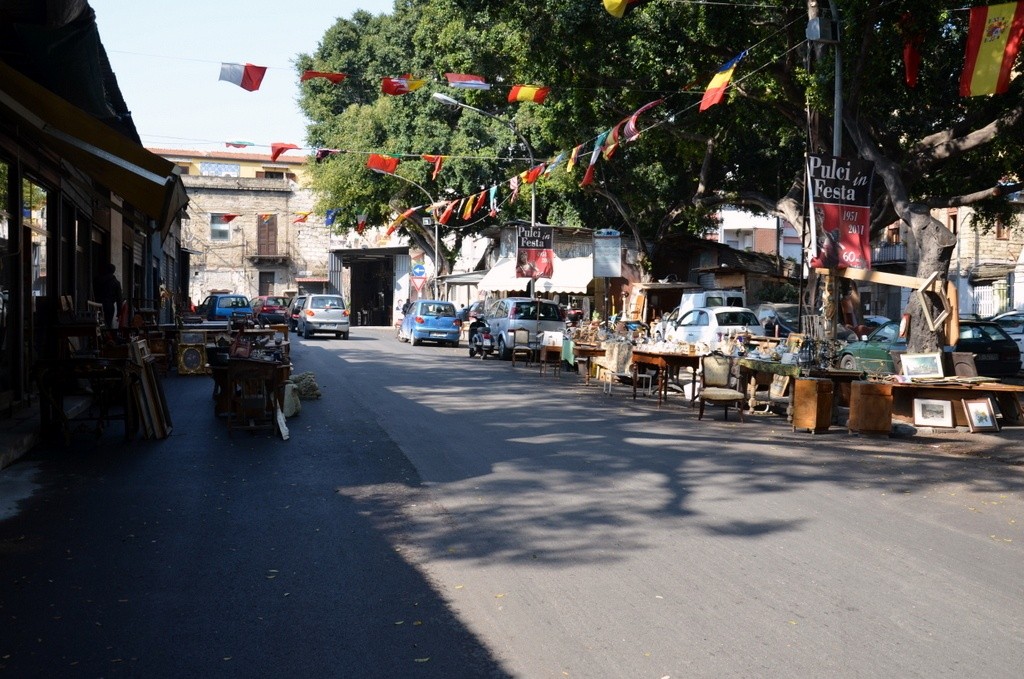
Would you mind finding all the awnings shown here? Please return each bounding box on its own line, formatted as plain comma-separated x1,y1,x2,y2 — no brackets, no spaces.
478,257,593,294
0,60,190,247
295,278,328,282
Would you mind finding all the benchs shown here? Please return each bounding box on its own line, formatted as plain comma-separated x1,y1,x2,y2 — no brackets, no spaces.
603,371,652,397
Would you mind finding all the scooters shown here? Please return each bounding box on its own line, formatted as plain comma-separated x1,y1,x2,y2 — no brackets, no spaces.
467,314,493,360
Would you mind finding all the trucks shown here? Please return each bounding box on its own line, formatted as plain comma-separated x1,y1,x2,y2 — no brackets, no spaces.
655,291,746,342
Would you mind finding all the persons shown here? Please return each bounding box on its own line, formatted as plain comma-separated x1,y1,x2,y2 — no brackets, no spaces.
402,299,411,315
396,299,403,310
104,264,122,328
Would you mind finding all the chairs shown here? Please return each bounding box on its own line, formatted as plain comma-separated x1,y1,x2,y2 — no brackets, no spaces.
696,350,745,424
512,327,534,367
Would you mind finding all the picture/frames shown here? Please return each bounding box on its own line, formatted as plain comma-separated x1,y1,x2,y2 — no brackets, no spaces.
912,397,954,429
770,333,807,397
961,396,1000,433
899,351,945,378
917,271,952,332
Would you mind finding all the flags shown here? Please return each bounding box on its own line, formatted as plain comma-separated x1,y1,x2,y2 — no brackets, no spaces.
960,0,1024,96
700,52,744,113
218,61,663,238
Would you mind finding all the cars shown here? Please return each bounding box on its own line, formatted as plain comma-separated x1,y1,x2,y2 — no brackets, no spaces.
197,292,253,323
664,305,765,344
486,296,567,359
249,295,295,325
747,303,858,341
960,310,1024,371
456,299,485,324
285,293,350,339
839,318,1021,378
397,299,459,346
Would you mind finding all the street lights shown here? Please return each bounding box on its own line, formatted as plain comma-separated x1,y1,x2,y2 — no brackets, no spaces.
433,90,535,296
371,167,439,312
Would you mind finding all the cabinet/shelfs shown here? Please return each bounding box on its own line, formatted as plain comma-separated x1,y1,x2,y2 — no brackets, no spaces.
846,379,893,435
792,377,833,435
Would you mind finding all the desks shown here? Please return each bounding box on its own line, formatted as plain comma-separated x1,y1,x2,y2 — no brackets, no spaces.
537,333,1024,437
37,320,292,448
